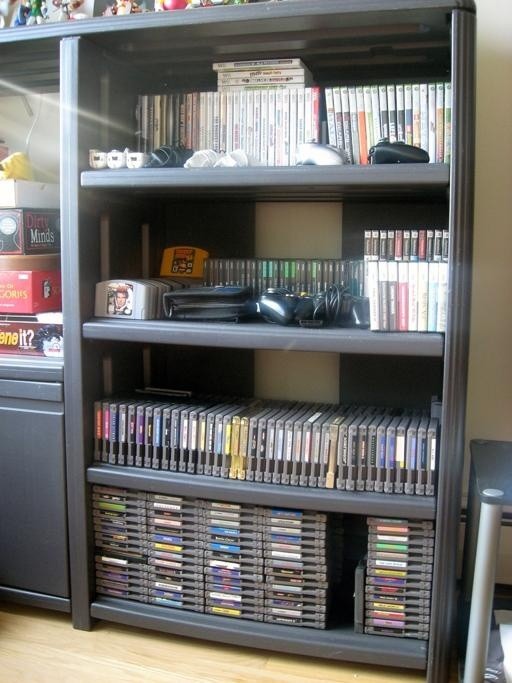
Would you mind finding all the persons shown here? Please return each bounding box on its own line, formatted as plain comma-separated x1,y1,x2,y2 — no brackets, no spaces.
107,286,132,316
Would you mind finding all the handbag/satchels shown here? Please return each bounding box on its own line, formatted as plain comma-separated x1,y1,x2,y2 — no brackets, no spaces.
162,287,250,322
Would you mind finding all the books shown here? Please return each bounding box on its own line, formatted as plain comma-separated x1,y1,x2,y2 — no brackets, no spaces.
364,261,450,333
135,58,452,165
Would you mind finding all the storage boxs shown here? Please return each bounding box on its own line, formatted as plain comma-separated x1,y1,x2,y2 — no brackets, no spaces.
0,320,64,357
0,177,60,207
1,255,61,314
0,208,61,255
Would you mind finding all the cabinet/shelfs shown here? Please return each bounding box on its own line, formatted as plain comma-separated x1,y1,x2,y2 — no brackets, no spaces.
0,0,477,683
